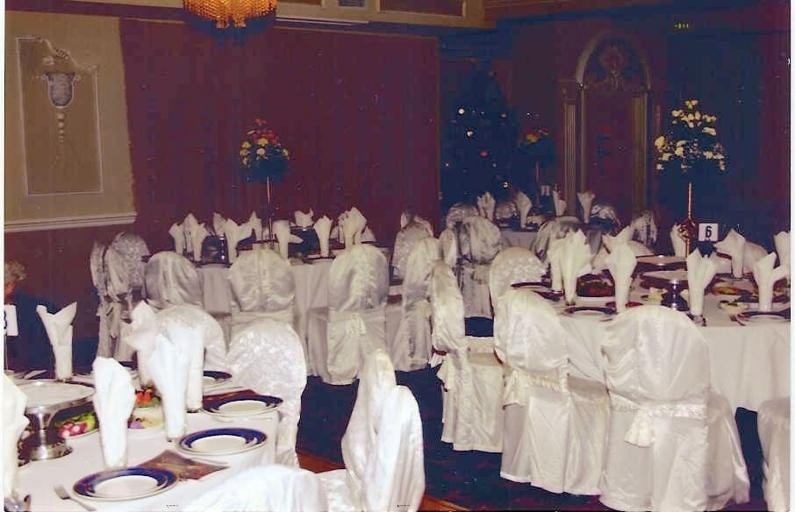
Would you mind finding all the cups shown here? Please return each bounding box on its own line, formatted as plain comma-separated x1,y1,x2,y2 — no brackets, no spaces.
53,344,72,383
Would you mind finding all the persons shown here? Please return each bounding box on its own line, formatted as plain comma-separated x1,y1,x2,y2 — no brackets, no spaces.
4,259,62,369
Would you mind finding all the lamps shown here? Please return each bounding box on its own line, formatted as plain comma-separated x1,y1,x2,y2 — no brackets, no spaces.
182,1,278,35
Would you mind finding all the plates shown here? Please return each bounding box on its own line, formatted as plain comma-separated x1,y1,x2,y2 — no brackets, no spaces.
72,365,281,501
510,253,790,324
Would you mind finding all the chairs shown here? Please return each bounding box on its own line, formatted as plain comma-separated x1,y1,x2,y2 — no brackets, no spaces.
4,187,792,511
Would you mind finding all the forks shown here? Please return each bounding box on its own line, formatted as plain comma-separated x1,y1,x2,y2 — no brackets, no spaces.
52,482,98,512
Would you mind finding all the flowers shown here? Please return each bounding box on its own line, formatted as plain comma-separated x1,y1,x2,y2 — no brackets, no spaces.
650,93,737,181
239,116,294,185
